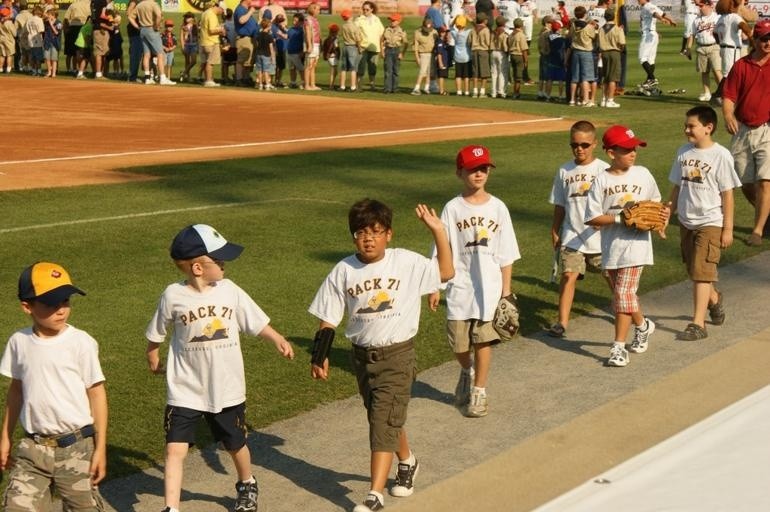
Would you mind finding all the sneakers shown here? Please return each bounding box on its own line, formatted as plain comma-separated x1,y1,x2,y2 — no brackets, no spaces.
698,93,712,101
391,456,419,496
235,475,257,512
680,48,691,60
353,494,381,512
462,391,487,417
747,232,762,246
455,368,476,403
546,292,725,366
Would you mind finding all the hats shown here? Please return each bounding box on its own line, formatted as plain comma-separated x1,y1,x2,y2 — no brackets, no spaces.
17,260,85,305
753,19,770,39
602,126,647,149
170,224,244,260
456,145,496,170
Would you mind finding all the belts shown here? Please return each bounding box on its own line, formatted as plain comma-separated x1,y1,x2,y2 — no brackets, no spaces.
695,42,717,46
720,45,740,49
24,423,94,449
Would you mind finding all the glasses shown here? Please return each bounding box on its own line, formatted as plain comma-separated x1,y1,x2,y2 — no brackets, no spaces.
569,142,591,148
354,229,385,238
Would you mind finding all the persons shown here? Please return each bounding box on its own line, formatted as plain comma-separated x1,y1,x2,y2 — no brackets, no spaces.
0,261,110,512
722,17,770,246
309,196,455,512
634,0,770,102
582,126,672,368
664,102,743,341
145,223,295,512
0,0,630,109
426,144,521,416
546,120,612,338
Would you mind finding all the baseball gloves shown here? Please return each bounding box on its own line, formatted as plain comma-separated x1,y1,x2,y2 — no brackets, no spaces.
621,200,670,232
493,292,521,342
